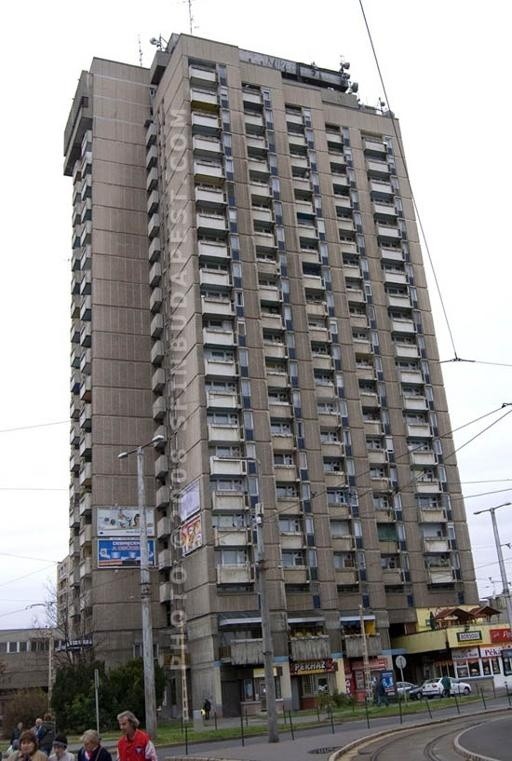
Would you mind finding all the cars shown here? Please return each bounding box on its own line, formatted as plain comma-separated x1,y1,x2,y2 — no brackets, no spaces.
421,677,472,700
384,681,423,703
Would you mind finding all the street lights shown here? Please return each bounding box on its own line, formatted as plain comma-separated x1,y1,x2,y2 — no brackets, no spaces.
116,434,168,739
474,501,512,633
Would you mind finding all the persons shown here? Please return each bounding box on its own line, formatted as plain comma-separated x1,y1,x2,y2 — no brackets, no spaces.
100,509,141,530
3,711,157,761
203,699,211,721
376,678,389,707
442,674,451,699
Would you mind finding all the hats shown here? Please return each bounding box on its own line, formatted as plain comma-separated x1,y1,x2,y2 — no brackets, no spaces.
20,730,100,747
